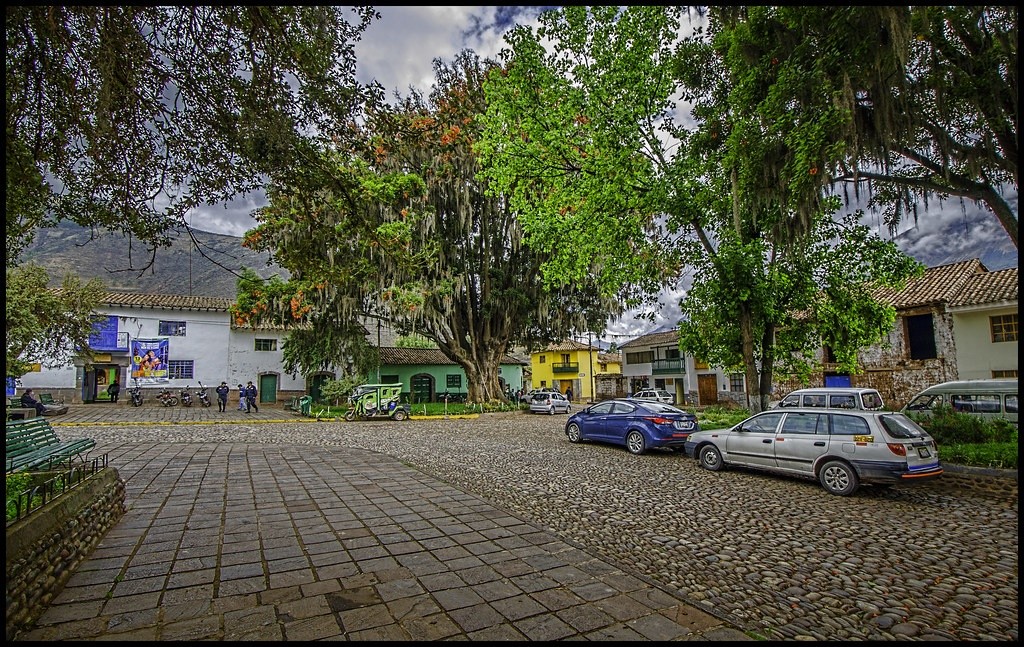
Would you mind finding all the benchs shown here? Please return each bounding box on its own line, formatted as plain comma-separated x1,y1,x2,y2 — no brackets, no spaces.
5,417,98,479
39,393,65,406
8,397,23,409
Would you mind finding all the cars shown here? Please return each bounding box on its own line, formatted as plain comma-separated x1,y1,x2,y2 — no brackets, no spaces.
684,409,944,497
565,398,700,454
530,391,572,415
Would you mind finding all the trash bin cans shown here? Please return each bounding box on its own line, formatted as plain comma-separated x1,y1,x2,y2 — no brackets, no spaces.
515,396,517,402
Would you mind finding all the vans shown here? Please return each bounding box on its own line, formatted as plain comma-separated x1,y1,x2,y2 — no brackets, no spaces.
901,379,1018,427
771,387,885,411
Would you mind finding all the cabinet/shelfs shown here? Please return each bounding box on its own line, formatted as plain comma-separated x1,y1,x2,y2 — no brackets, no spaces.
284,395,298,410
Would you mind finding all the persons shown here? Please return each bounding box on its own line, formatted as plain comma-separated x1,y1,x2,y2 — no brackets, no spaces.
107,380,119,403
216,382,230,412
21,388,52,415
509,388,524,403
245,381,259,413
566,387,572,402
139,350,169,371
237,384,248,410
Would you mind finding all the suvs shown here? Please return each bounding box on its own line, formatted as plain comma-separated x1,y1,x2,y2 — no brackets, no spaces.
521,387,564,404
631,390,675,405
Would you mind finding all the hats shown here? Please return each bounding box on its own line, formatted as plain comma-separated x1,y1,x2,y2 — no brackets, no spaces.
222,382,226,384
114,380,117,382
238,384,242,387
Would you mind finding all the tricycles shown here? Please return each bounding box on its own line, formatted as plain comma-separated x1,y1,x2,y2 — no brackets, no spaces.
343,383,407,421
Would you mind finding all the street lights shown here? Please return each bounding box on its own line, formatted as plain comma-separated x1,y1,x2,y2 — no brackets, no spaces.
575,335,594,403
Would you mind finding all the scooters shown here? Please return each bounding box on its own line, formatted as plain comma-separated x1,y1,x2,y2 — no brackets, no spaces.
126,377,212,407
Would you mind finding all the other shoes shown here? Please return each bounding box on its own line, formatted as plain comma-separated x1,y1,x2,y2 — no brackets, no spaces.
256,408,258,412
245,411,250,413
44,410,51,413
223,410,225,412
219,409,222,412
